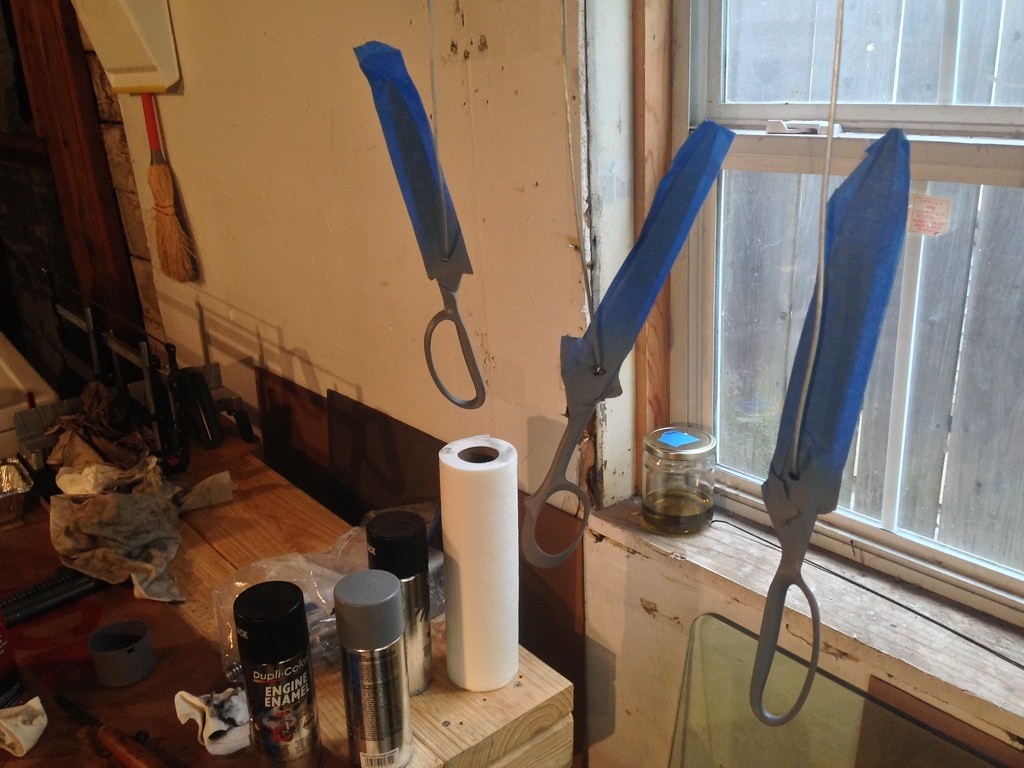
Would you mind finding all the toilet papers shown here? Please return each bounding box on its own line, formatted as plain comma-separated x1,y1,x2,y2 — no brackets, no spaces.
439,434,520,693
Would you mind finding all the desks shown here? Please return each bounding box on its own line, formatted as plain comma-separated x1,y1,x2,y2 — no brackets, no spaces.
0,413,574,768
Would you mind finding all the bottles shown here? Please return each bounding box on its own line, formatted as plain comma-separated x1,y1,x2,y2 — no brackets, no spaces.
334,571,414,768
366,512,432,695
233,581,315,768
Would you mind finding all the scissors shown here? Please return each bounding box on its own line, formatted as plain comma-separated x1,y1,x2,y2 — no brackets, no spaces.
355,41,487,411
518,121,734,571
750,127,911,728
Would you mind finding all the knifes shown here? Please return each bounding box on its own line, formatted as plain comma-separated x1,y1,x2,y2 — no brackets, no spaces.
54,693,167,768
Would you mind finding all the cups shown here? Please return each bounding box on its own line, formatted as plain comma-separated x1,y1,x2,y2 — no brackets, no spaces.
90,620,154,690
639,428,718,533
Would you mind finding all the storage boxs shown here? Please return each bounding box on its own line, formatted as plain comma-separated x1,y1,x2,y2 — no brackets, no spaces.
0,332,60,460
